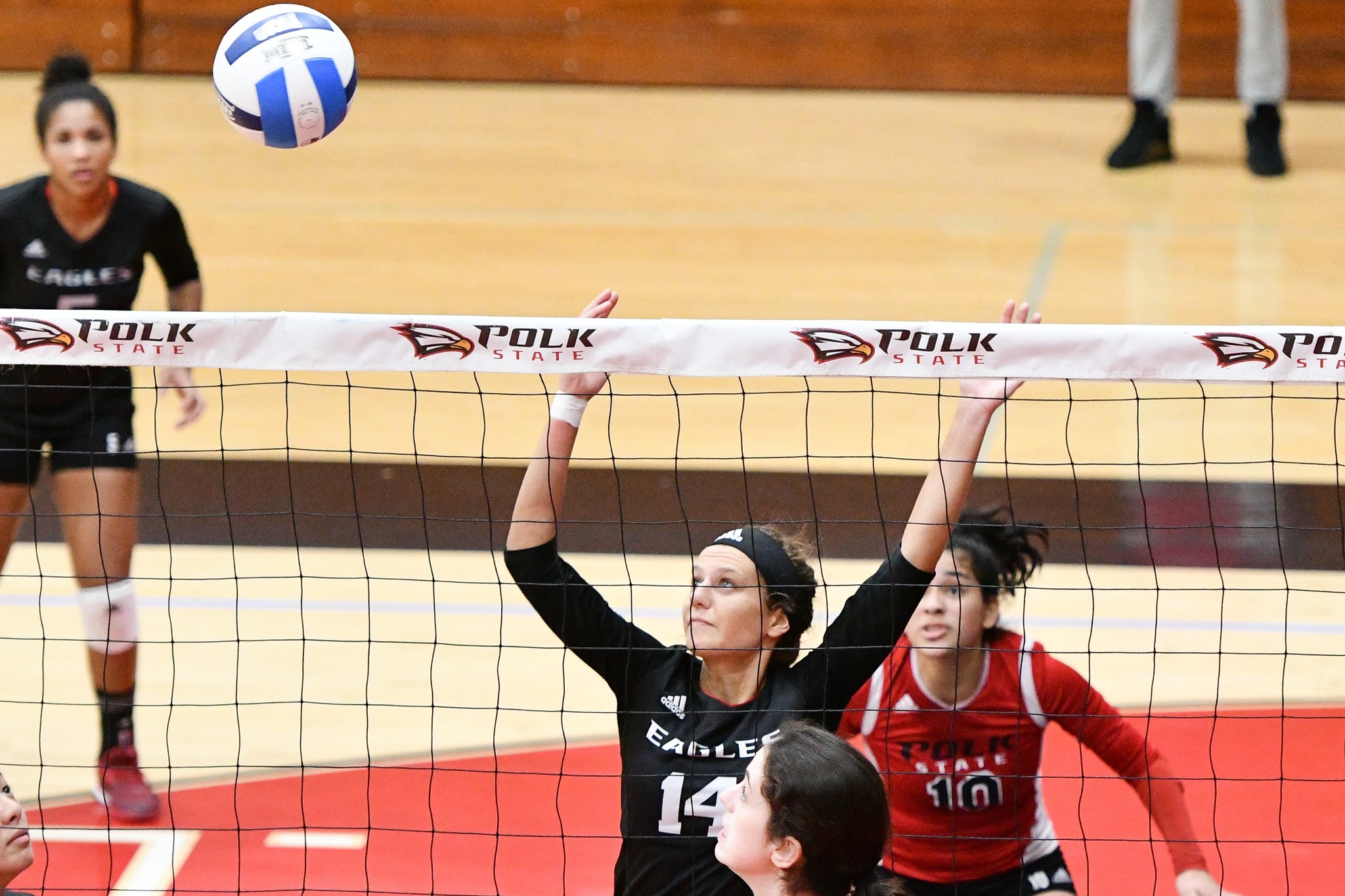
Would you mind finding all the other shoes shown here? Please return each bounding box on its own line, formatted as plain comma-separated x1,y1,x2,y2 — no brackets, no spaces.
1243,102,1287,174
93,745,159,821
1108,97,1175,168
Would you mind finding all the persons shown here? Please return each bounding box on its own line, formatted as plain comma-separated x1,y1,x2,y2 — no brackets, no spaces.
837,502,1239,896
502,288,1044,896
0,54,207,821
1103,0,1291,176
0,771,36,896
714,718,905,896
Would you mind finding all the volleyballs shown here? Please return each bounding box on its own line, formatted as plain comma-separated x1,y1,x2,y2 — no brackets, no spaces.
213,4,357,149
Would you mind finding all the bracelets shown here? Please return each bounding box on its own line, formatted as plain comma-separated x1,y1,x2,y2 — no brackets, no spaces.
550,389,589,427
